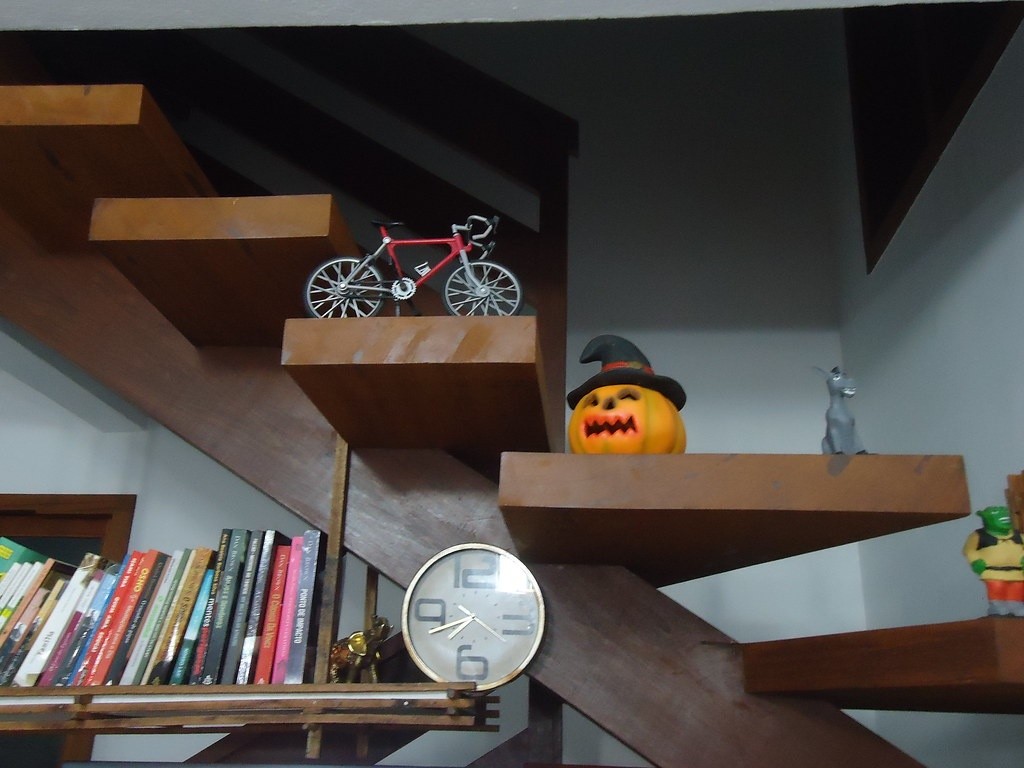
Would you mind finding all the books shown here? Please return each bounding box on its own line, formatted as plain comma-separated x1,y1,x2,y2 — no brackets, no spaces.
0,526,326,685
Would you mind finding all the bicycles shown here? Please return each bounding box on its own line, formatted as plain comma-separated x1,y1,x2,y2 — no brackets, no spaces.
303,214,524,319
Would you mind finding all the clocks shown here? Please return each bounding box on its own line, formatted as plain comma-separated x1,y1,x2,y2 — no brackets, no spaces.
400,541,545,691
